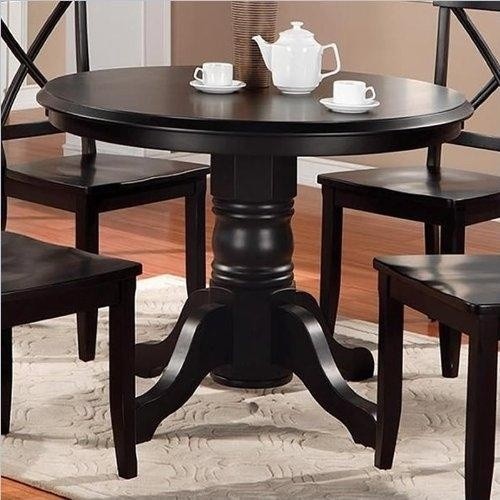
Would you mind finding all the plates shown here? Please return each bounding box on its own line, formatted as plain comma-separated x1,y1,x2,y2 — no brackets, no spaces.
190,78,247,94
319,97,381,114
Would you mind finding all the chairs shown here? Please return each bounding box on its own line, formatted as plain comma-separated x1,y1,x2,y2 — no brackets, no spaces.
371,254,499,497
1,0,210,362
315,2,500,378
1,227,144,481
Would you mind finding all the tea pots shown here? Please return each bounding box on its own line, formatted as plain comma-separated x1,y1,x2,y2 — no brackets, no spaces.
249,20,341,96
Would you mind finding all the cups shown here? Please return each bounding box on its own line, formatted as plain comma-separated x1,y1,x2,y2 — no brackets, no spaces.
333,79,376,105
194,62,234,86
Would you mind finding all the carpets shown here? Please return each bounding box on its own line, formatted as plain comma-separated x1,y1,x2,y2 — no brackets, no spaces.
1,272,500,500
